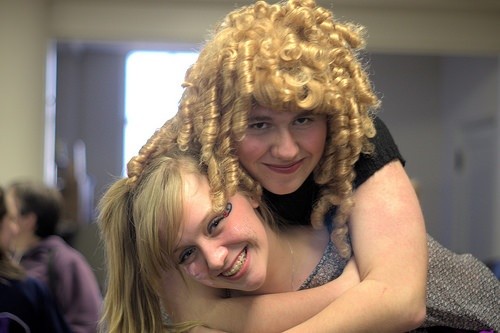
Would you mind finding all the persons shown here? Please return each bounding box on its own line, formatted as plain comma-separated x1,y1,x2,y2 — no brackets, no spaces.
10,181,104,333
0,187,66,333
139,0,431,333
94,147,500,333
